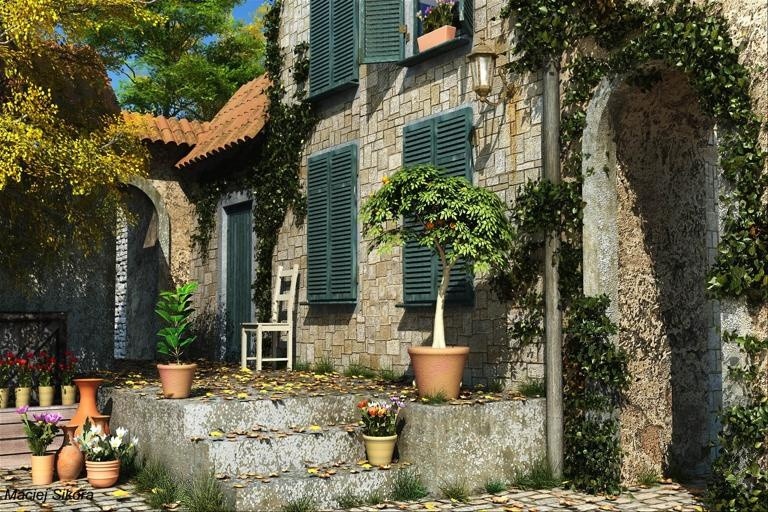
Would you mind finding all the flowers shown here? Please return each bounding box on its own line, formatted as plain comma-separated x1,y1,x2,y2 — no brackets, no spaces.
60,350,77,386
357,396,406,436
415,0,454,34
75,416,139,461
12,352,33,385
16,406,62,456
35,352,57,385
0,350,12,385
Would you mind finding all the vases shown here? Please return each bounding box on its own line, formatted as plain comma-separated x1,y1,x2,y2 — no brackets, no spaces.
71,379,110,442
56,425,84,482
91,416,109,432
0,389,9,409
31,454,55,484
362,434,399,466
85,460,120,488
61,386,76,406
39,386,53,406
416,25,456,52
15,388,31,407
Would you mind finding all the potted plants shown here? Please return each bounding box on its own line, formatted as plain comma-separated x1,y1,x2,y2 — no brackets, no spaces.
360,166,515,396
156,281,197,398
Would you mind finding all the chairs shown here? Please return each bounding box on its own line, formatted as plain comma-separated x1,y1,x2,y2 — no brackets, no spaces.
241,265,299,371
